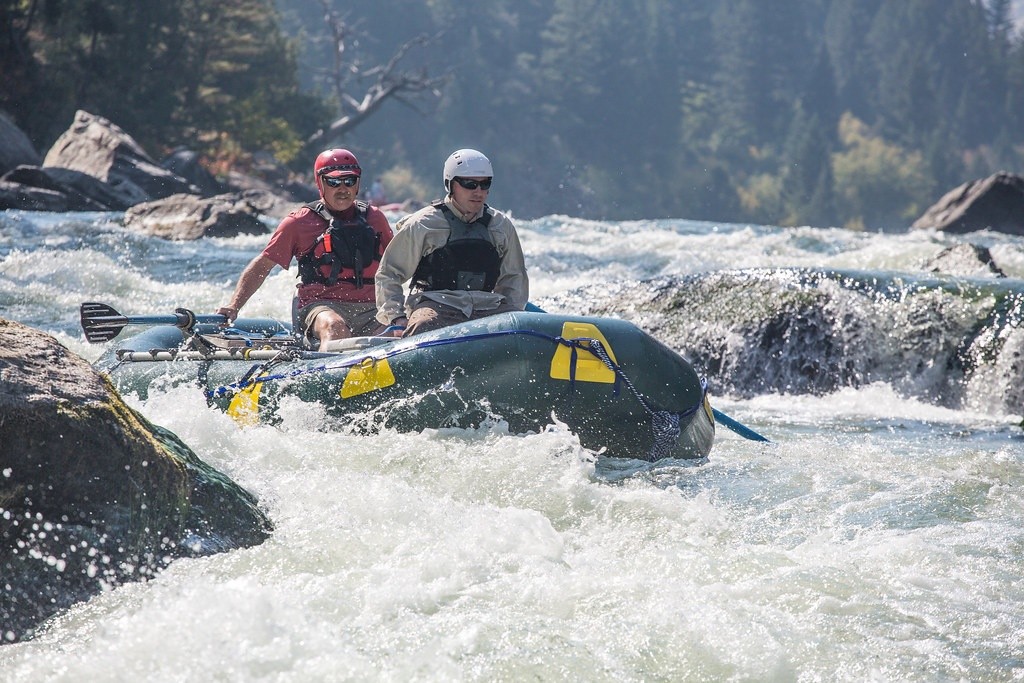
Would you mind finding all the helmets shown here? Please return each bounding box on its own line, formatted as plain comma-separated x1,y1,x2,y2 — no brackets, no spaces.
315,148,361,199
443,149,494,195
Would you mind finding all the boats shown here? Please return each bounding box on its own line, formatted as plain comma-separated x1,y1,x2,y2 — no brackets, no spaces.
90,312,716,472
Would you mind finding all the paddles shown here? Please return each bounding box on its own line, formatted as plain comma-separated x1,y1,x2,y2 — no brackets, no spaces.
80,300,229,346
522,300,768,443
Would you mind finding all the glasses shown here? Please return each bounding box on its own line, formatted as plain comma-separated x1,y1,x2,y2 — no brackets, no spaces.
325,176,357,189
455,177,492,190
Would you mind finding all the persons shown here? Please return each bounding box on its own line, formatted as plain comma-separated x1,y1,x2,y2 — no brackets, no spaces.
215,149,396,353
374,147,531,337
